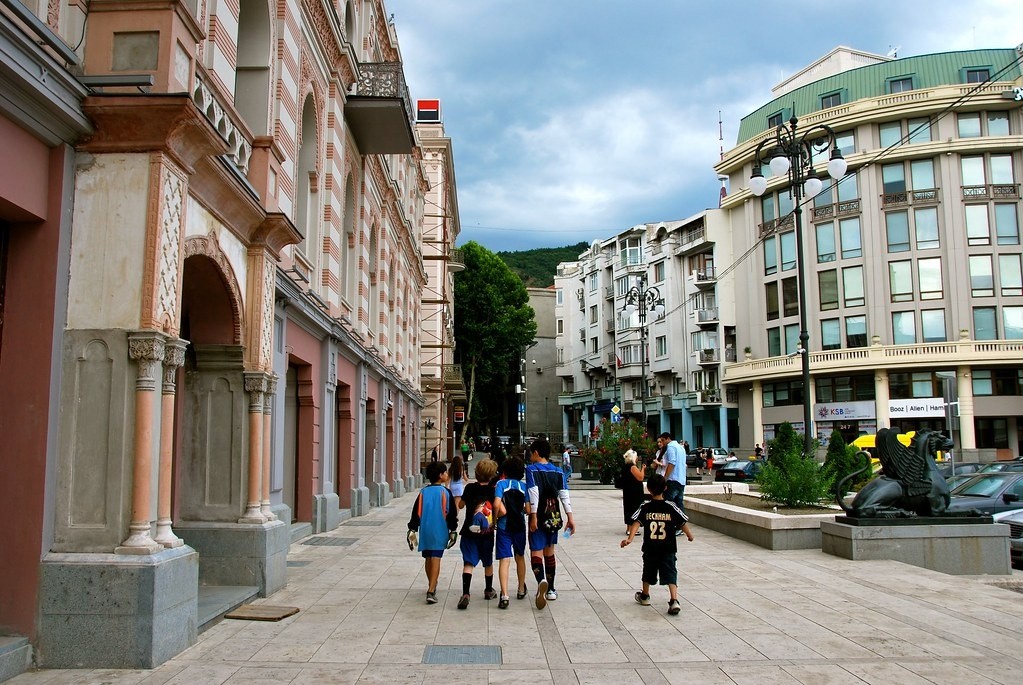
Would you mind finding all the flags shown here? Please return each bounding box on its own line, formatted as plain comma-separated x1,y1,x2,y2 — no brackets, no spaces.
617,358,621,367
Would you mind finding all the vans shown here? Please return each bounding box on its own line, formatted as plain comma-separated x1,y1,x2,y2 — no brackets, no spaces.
849,432,944,490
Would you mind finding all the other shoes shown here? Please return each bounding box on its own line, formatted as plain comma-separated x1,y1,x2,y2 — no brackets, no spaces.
697,472,706,475
626,530,641,536
708,472,713,476
675,529,685,536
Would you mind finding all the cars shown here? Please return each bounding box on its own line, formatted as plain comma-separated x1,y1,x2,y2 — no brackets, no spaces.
715,459,769,483
523,436,539,447
686,447,729,468
937,458,1023,564
479,436,491,446
552,442,583,453
497,435,514,448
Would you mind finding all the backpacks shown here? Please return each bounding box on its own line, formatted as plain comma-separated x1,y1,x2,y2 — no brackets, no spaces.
468,484,494,534
535,466,562,534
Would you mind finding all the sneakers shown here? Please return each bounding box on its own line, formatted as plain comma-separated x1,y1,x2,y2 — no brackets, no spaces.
517,583,527,599
536,579,557,610
484,588,496,601
667,598,681,615
457,594,470,610
426,589,438,603
498,593,509,609
634,591,652,606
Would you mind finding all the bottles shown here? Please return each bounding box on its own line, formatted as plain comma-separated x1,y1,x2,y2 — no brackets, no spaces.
563,529,570,539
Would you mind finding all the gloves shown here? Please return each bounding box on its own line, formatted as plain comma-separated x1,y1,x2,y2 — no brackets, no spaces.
406,530,418,551
447,532,457,549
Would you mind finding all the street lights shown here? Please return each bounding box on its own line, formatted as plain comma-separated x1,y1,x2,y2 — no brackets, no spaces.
620,284,665,432
750,104,848,456
424,421,434,467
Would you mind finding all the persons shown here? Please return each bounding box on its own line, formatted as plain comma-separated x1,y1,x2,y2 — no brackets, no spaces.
562,447,572,482
407,461,459,604
457,459,506,610
725,451,737,463
695,447,716,476
622,449,647,535
679,440,690,454
444,456,469,515
460,437,476,463
620,474,694,615
493,457,531,609
431,447,439,462
755,443,766,461
650,434,668,476
660,431,688,536
525,438,575,610
